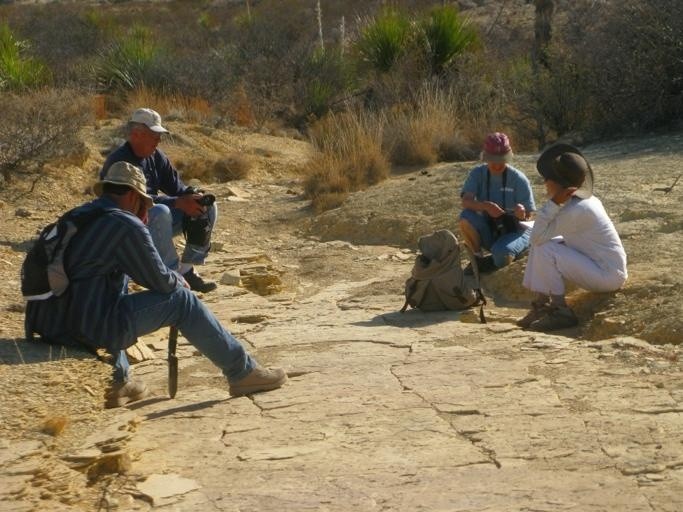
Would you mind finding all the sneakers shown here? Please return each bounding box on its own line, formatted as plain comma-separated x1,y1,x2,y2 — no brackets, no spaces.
229,365,288,396
530,304,578,329
481,256,493,265
109,381,148,407
517,302,544,327
182,268,216,293
465,256,484,275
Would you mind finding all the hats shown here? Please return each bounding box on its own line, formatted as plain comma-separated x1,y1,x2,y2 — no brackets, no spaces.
130,108,169,135
537,144,594,199
484,133,512,161
94,161,153,208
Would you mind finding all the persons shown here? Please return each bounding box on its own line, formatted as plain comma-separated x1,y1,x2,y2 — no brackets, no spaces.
517,143,629,330
456,131,534,276
98,107,219,293
20,161,288,408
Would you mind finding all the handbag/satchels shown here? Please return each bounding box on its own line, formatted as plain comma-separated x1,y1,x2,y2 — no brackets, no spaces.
492,214,516,242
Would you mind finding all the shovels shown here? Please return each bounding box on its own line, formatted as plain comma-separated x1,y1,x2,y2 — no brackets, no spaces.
167,325,178,399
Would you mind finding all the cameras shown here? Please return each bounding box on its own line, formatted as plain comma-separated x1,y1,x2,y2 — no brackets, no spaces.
198,194,216,206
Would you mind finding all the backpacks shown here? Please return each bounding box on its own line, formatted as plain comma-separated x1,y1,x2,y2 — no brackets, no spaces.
21,203,136,304
400,230,486,323
